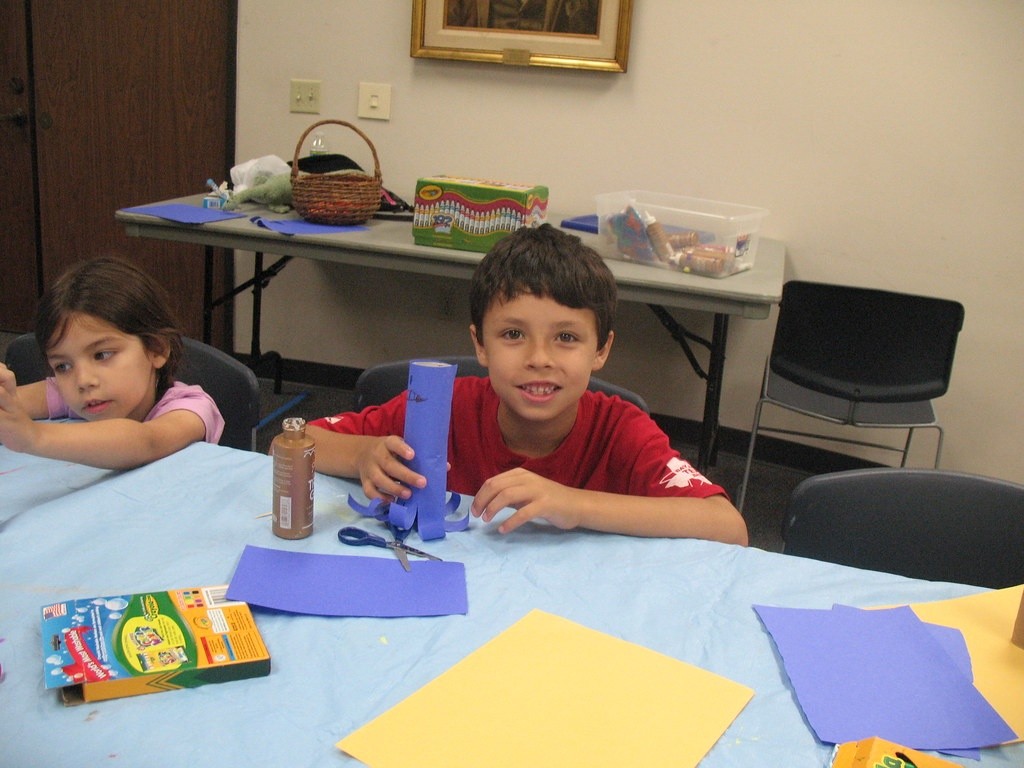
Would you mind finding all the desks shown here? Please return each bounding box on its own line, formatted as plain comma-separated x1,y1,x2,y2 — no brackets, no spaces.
113,186,785,481
0,417,1024,768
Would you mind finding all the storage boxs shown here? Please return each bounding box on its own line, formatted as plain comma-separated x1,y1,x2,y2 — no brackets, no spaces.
412,175,550,253
39,586,270,707
588,191,772,281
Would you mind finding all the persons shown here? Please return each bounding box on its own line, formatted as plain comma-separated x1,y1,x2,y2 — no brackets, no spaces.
0,254,226,471
302,223,750,547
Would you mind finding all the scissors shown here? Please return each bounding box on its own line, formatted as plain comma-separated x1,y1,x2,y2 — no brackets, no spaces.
337,509,443,573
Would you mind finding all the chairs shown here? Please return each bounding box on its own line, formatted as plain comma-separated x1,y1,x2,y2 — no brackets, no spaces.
777,466,1024,591
349,354,650,419
3,330,259,454
731,280,965,516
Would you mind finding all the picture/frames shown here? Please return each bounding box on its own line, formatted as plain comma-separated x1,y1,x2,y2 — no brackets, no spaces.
410,0,635,73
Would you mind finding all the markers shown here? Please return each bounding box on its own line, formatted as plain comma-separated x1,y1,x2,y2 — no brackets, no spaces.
414,198,526,234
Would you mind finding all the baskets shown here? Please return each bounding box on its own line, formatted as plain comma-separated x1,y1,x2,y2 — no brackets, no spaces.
290,119,382,225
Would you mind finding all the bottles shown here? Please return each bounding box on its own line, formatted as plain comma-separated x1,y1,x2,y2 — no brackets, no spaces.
309,131,328,155
271,418,316,540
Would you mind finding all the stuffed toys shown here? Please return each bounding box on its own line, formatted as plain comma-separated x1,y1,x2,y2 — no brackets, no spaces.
222,168,364,213
